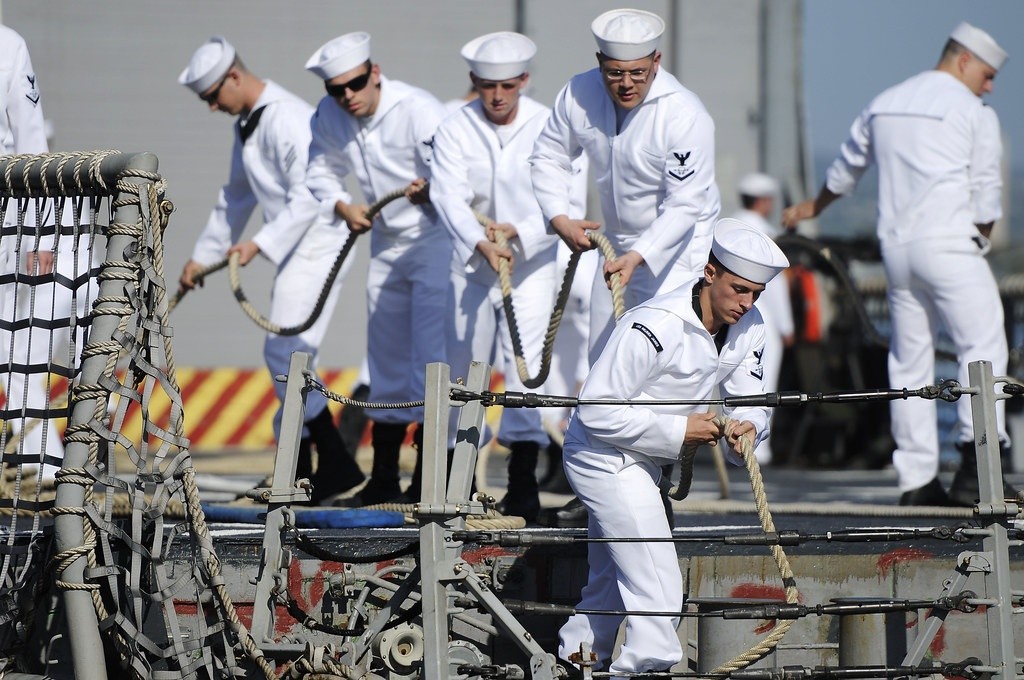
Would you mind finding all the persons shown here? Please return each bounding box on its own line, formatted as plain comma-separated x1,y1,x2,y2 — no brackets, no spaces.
781,21,1024,506
431,31,570,524
532,7,722,522
539,241,600,495
557,218,789,680
1,25,100,483
731,174,796,467
177,37,367,504
339,84,483,457
303,32,453,503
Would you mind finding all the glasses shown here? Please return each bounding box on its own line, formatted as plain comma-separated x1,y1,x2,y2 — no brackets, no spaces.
202,71,231,104
601,65,654,81
324,61,371,98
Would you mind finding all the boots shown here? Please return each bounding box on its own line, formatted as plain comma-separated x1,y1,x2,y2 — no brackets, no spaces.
535,487,592,528
900,473,950,507
332,423,406,507
386,426,423,504
297,439,312,482
488,439,541,519
302,406,365,505
947,441,1022,506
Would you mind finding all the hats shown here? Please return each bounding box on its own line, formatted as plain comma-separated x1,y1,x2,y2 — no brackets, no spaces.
591,7,664,61
738,173,777,199
179,35,236,96
711,217,790,285
304,31,372,82
460,32,537,82
950,23,1009,72
